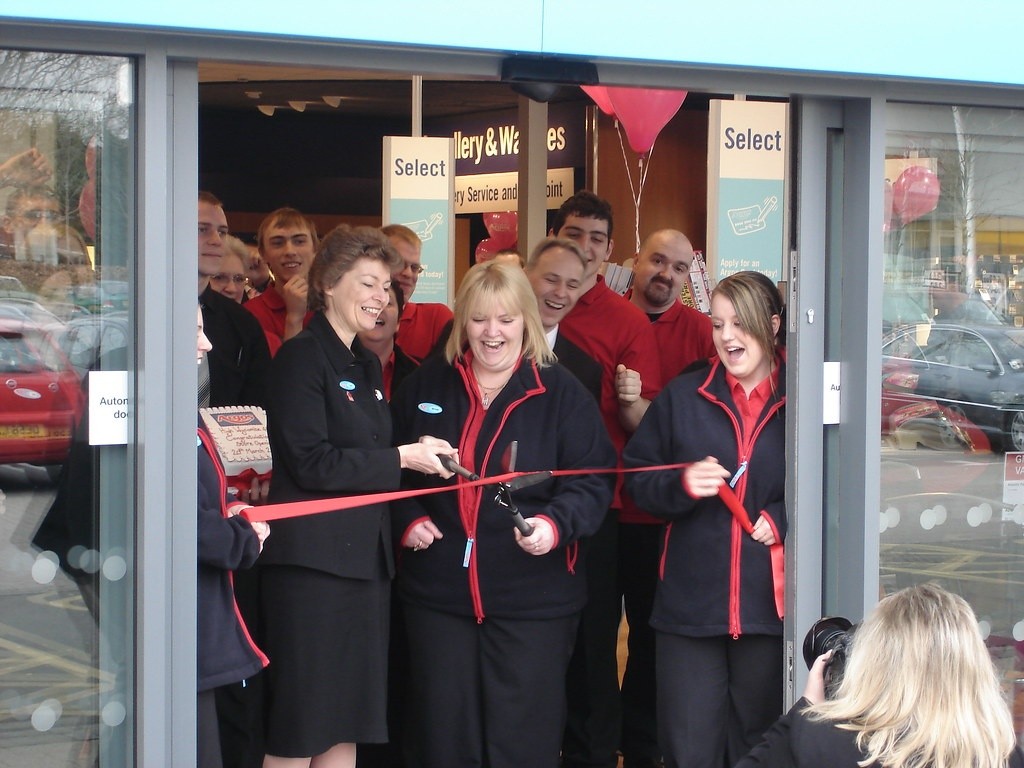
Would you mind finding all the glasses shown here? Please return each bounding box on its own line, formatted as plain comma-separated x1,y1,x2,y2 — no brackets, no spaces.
16,209,59,222
405,263,423,273
210,272,249,287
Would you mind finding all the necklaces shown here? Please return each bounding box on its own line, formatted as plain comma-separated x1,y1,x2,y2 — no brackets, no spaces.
474,366,512,406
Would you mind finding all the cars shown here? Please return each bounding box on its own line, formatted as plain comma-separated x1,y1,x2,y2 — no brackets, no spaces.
883,284,1024,459
0,279,131,481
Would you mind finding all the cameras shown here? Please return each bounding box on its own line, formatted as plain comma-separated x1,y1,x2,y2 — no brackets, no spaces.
803,617,863,701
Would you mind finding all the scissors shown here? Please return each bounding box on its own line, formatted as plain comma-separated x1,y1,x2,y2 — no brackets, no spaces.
435,441,553,538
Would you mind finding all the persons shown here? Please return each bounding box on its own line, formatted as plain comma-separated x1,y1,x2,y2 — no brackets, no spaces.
735,583,1024,768
0,145,787,768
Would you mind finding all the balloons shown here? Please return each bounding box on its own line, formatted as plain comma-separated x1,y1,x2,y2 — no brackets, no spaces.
579,86,688,152
884,166,939,233
80,134,96,240
475,212,518,263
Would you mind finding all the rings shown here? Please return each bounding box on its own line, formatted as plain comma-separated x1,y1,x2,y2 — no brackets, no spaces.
414,541,423,551
